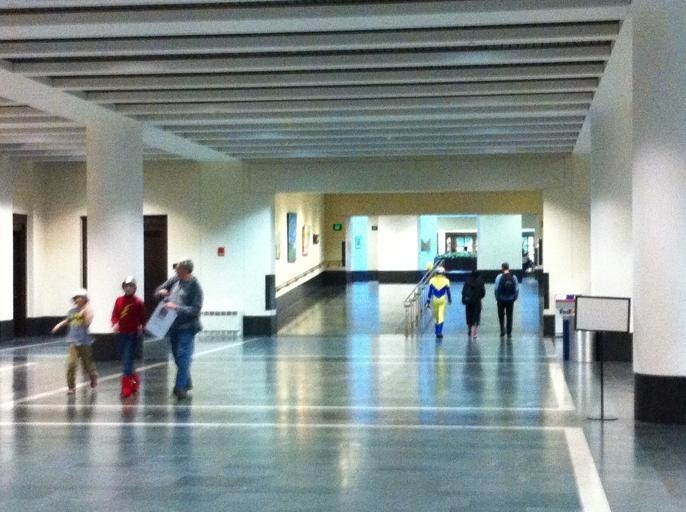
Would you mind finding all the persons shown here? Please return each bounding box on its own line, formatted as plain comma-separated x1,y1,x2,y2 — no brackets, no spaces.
522,252,532,277
426,265,452,338
152,259,203,401
50,288,98,396
494,262,519,337
461,269,487,338
110,276,146,398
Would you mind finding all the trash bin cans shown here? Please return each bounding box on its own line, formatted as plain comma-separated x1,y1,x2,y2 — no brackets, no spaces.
568,315,597,364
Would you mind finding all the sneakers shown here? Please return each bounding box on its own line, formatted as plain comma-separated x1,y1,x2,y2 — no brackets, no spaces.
173,385,192,395
177,387,186,402
435,326,512,337
67,386,76,394
89,375,97,388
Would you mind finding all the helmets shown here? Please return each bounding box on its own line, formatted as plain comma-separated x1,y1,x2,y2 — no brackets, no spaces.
435,266,445,274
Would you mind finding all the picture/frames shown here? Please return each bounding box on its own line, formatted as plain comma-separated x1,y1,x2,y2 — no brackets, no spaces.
286,211,299,265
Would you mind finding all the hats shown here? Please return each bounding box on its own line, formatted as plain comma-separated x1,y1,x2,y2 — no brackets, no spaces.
124,275,137,286
71,289,88,305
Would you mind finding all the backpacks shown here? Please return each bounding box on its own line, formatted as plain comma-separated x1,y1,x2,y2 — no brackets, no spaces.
497,271,516,296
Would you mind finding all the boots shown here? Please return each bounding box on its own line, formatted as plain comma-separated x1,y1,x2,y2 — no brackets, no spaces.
131,373,140,392
119,376,131,398
